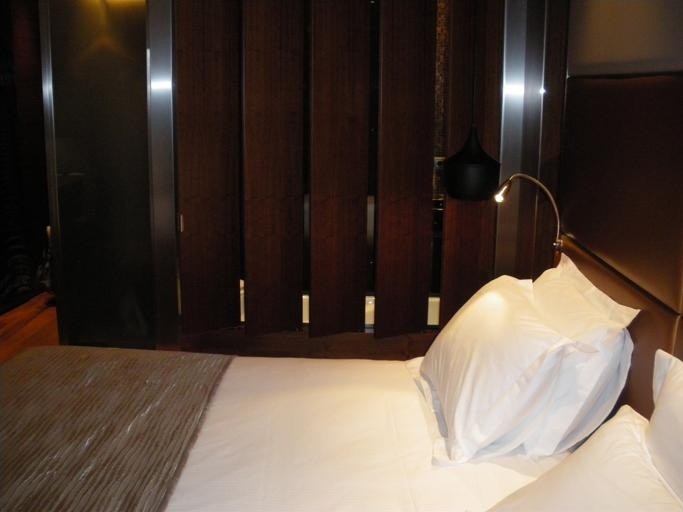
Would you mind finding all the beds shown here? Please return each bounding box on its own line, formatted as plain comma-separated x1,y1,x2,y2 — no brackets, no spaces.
0,74,683,512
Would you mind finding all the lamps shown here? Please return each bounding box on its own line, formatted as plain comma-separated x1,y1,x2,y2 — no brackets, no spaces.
438,0,500,202
494,172,563,250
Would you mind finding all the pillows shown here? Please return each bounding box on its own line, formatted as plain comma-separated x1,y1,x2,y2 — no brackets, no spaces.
405,251,641,466
486,346,682,510
